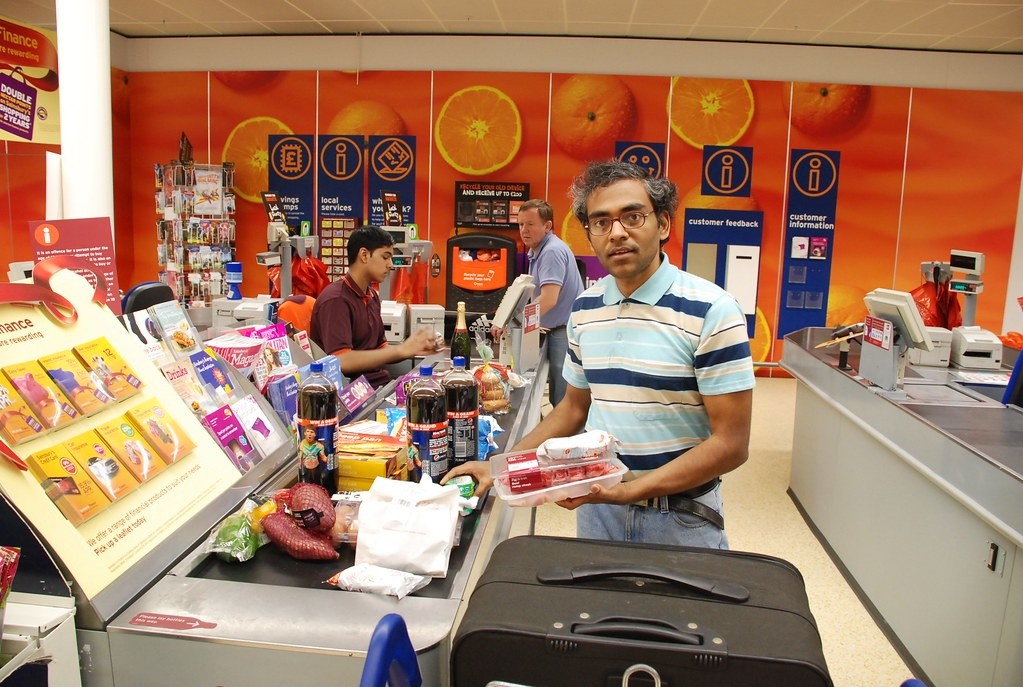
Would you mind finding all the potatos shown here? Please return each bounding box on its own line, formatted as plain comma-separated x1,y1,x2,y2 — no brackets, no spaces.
259,484,340,559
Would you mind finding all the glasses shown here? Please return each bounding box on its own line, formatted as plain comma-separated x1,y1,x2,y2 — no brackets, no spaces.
585,210,656,236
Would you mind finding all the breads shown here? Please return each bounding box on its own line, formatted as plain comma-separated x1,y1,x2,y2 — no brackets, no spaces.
478,373,509,414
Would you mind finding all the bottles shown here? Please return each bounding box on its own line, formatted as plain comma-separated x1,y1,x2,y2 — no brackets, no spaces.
406,365,448,487
442,356,479,473
499,323,512,366
450,301,471,371
296,359,341,506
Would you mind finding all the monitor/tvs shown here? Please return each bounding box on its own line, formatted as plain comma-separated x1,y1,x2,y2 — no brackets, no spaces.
864,288,934,354
490,274,536,329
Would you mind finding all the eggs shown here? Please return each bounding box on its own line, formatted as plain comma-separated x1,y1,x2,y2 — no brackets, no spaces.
327,505,358,549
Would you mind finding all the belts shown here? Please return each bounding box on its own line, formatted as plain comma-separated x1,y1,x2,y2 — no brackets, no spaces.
647,475,725,530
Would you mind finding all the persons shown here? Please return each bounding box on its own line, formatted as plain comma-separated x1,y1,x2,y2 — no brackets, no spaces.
263,346,283,374
406,426,422,482
436,156,757,551
308,225,437,392
490,198,586,409
299,423,328,486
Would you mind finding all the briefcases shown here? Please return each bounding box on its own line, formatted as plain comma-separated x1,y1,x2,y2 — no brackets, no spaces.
450,535,835,687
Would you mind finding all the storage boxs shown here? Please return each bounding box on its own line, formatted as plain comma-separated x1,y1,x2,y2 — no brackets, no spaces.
0,335,198,524
489,447,618,494
493,457,630,508
337,431,409,489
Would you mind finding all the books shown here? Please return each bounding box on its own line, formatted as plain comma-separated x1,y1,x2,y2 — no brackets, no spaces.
117,299,202,369
25,397,198,528
0,335,146,447
202,391,284,476
160,308,236,414
